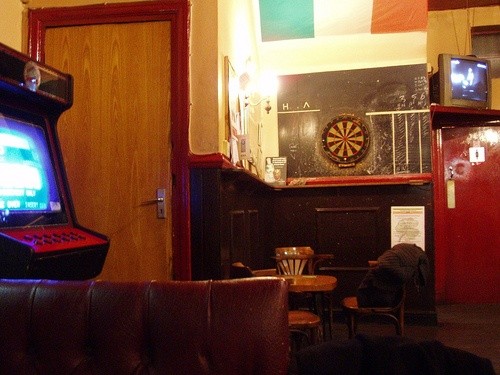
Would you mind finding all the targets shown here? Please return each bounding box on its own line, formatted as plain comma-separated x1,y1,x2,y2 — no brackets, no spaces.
320,113,371,164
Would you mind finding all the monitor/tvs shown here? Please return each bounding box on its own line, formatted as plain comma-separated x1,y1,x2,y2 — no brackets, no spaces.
0,111,68,228
428,54,492,109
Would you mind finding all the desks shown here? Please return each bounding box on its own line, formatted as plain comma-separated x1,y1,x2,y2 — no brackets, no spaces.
319,266,375,339
259,276,338,344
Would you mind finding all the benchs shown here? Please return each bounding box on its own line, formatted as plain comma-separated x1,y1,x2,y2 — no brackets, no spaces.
1,277,288,375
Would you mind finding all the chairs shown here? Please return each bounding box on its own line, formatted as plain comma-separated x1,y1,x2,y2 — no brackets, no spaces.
229,261,321,350
274,247,319,330
343,244,424,339
